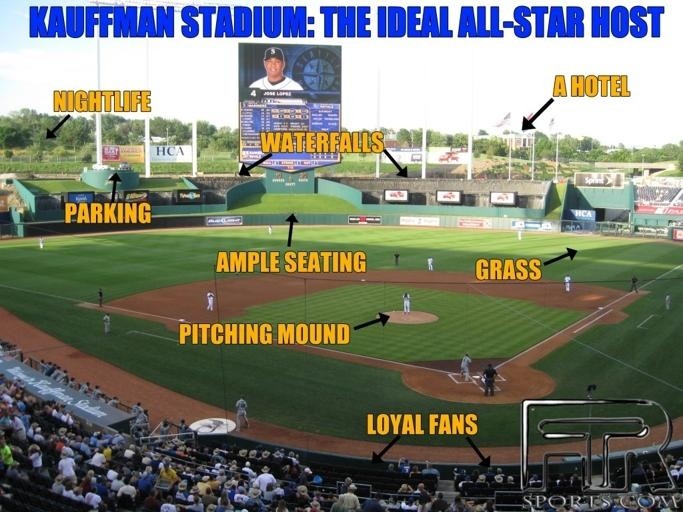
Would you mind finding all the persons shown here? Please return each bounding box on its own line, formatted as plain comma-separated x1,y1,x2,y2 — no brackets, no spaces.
629,274,638,293
252,440,682,509
401,291,411,316
459,353,472,383
248,46,305,92
482,361,498,397
664,292,671,310
206,289,215,311
101,312,110,334
0,342,250,511
564,273,570,292
97,287,103,307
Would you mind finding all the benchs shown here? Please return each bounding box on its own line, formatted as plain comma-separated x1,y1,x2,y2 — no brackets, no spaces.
1,441,682,510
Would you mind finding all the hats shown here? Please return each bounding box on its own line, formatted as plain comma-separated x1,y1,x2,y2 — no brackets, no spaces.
347,484,357,489
179,448,320,512
264,47,284,60
